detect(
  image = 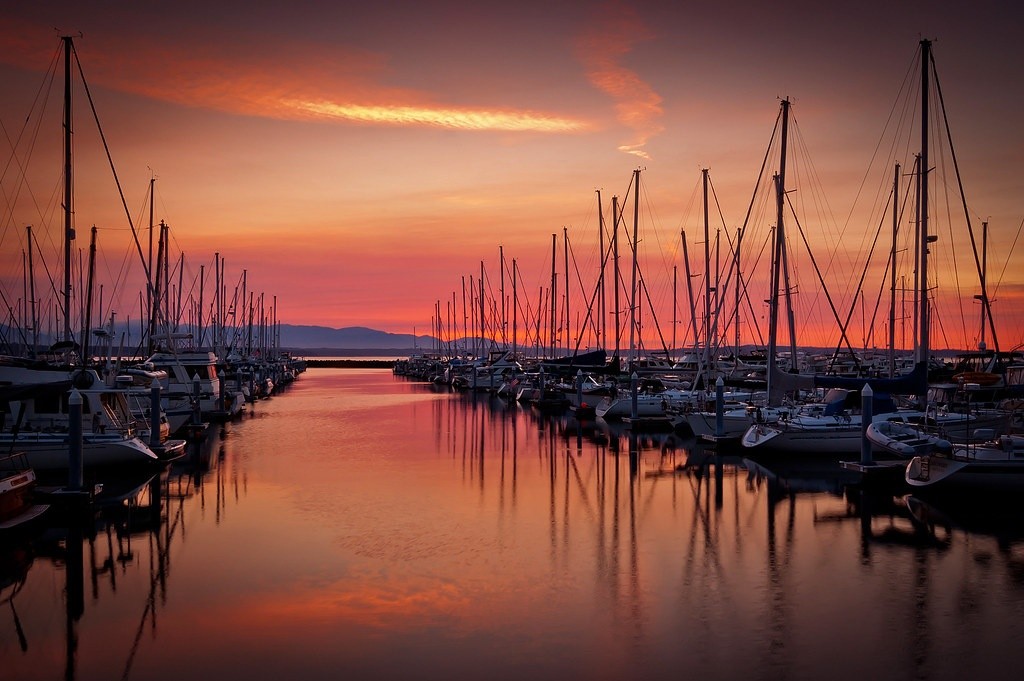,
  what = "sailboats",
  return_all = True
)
[393,31,1024,524]
[0,27,305,550]
